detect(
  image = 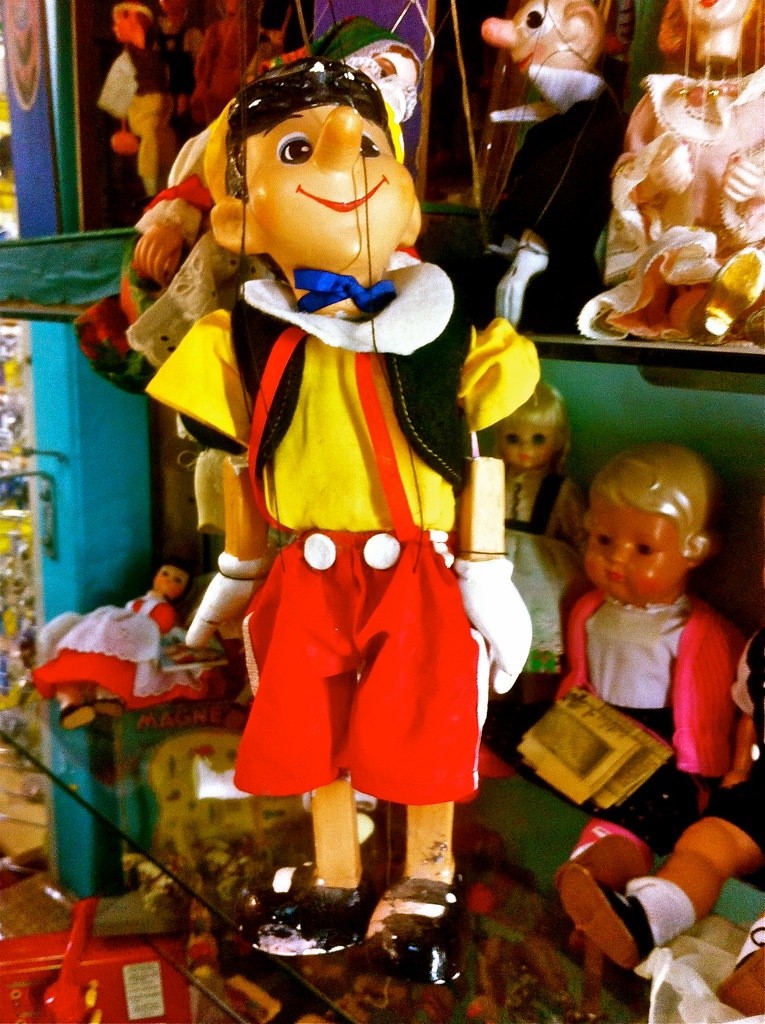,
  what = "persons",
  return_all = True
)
[33,0,764,989]
